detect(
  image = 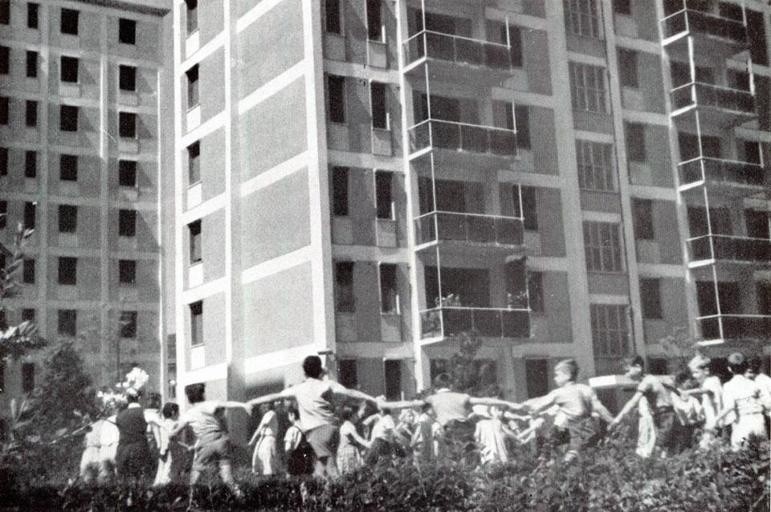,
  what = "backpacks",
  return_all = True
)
[286,425,319,477]
